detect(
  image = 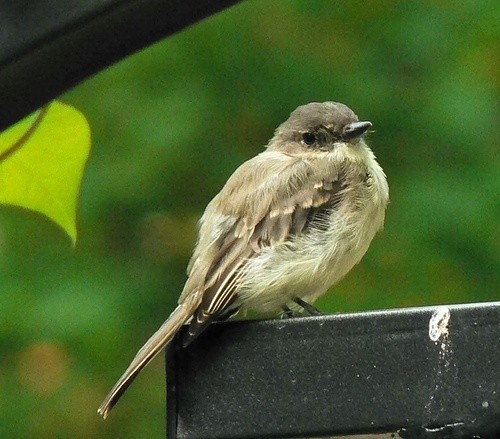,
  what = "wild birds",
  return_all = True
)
[98,100,391,422]
